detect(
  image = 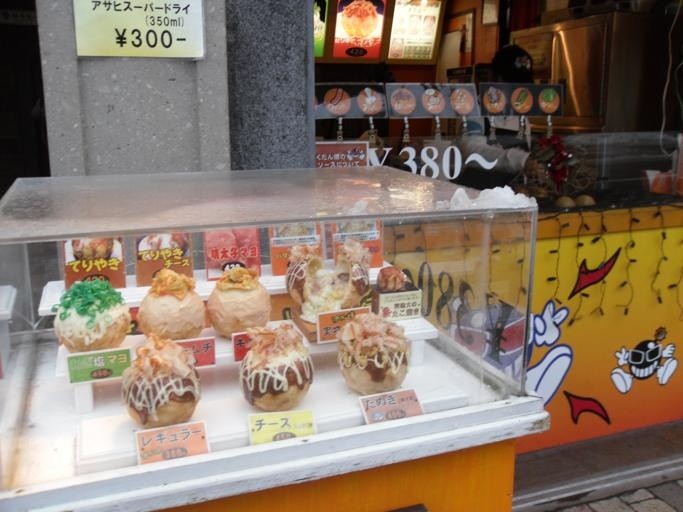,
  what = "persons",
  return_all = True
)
[454,44,548,191]
